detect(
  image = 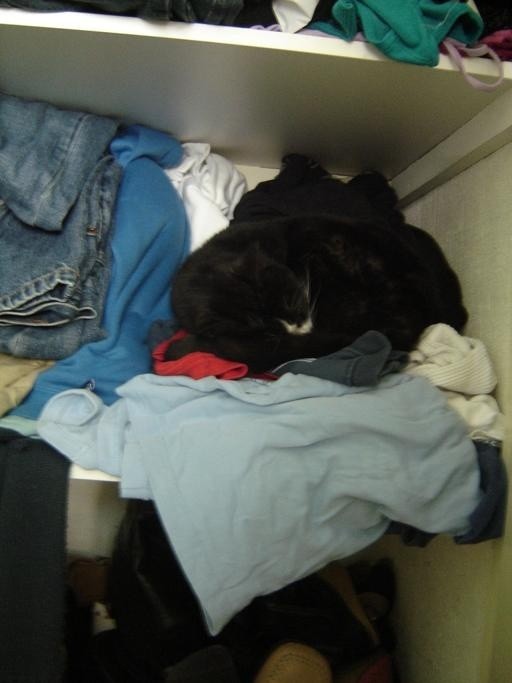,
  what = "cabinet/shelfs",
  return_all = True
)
[2,6,512,683]
